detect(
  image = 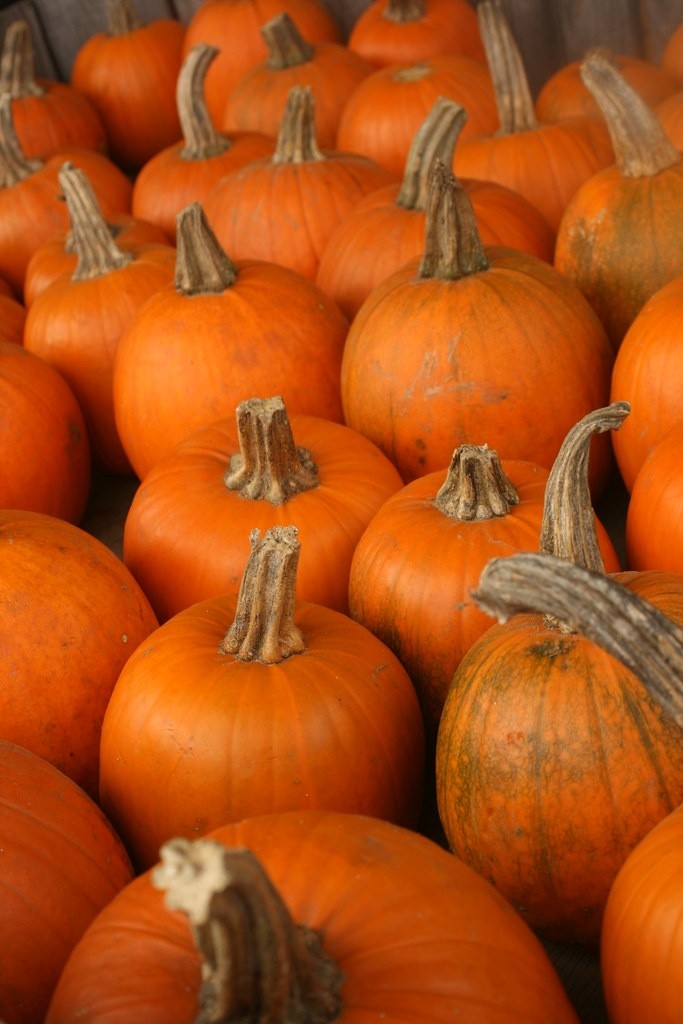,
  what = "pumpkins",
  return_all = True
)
[0,0,683,1024]
